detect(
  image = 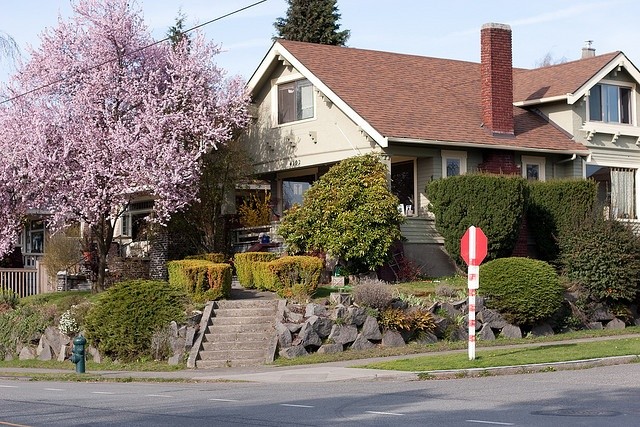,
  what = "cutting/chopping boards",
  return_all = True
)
[71,335,87,375]
